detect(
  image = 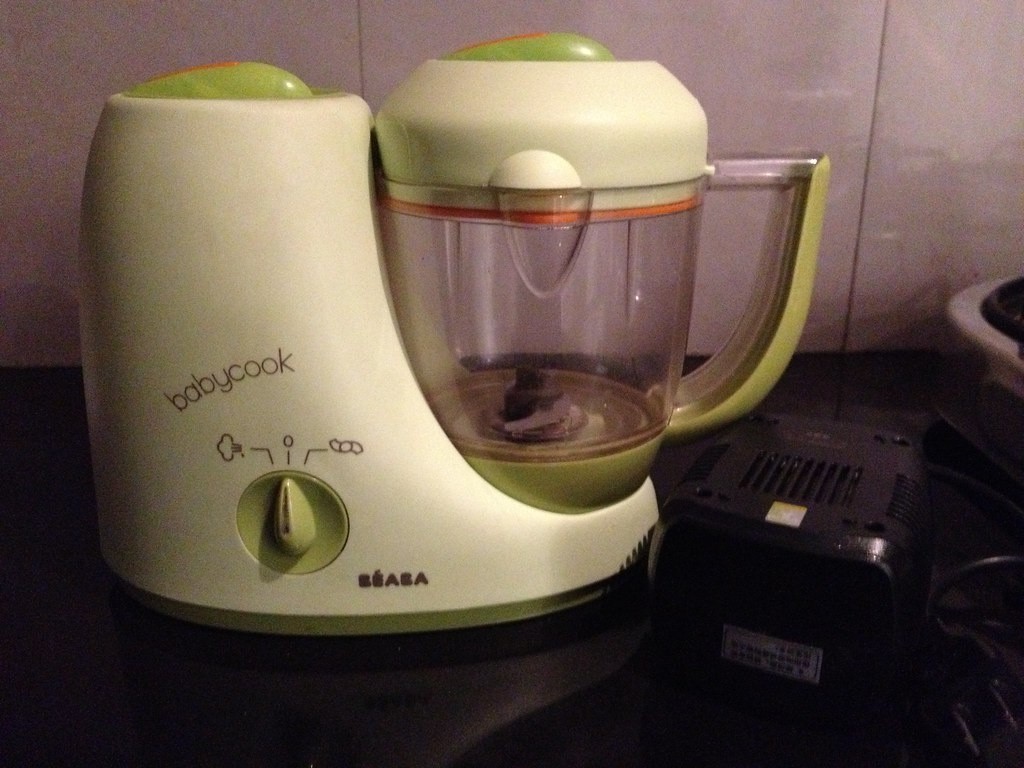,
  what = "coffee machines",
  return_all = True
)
[80,29,831,635]
